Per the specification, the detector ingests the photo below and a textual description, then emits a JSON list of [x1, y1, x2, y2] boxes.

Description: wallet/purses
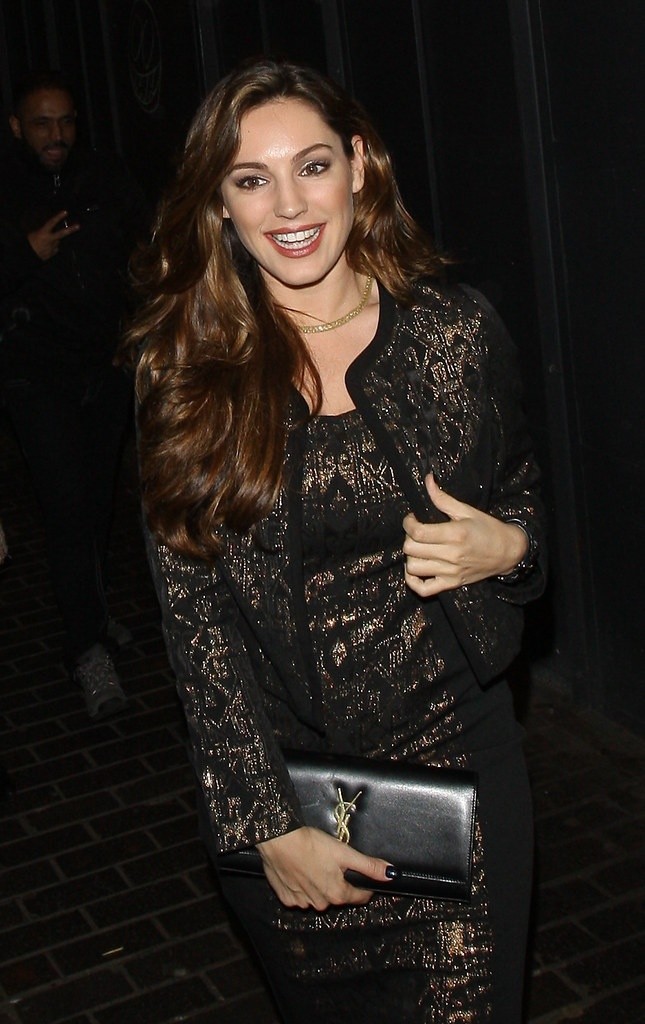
[[213, 750, 480, 906]]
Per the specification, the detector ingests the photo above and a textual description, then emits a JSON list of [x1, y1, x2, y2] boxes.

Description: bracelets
[[498, 517, 536, 584]]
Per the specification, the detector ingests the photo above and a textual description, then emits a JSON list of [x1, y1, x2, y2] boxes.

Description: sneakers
[[72, 640, 134, 724], [105, 616, 140, 649]]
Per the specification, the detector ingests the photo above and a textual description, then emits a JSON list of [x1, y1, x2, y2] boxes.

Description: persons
[[0, 65, 137, 720], [133, 60, 556, 1024]]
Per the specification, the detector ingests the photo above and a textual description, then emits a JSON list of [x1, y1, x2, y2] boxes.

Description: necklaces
[[295, 269, 373, 333]]
